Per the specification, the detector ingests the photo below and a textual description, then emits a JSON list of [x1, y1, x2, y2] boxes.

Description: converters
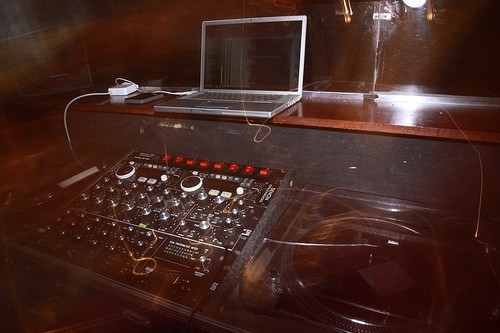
[[108, 83, 139, 96]]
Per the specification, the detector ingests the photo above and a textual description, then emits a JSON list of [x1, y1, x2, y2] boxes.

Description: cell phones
[[124, 90, 164, 104]]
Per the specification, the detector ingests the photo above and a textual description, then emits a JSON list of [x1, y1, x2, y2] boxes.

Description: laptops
[[154, 13, 306, 119]]
